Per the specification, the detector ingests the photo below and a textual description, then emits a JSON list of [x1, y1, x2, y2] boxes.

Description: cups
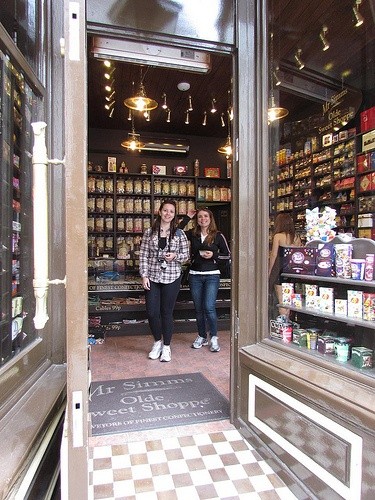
[[350, 259, 365, 280], [283, 323, 293, 342]]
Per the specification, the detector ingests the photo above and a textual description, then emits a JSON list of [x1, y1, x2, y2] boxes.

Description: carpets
[[88, 373, 232, 438]]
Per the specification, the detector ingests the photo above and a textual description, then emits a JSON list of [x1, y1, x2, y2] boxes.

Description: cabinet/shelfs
[[86, 125, 374, 382]]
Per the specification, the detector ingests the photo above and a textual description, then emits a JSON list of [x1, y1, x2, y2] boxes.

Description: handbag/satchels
[[181, 262, 193, 286]]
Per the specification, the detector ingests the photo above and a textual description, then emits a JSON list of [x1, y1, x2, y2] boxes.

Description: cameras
[[158, 259, 168, 269]]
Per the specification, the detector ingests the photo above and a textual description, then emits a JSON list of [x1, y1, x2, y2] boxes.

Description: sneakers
[[209, 336, 220, 352], [192, 334, 208, 348], [159, 344, 171, 362], [148, 339, 163, 359]]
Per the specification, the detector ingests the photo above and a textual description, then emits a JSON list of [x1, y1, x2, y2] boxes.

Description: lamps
[[122, 82, 143, 149], [218, 88, 234, 155], [293, 55, 305, 71], [272, 73, 281, 86], [318, 33, 332, 51], [352, 6, 365, 28], [268, 87, 289, 122], [104, 59, 233, 127]]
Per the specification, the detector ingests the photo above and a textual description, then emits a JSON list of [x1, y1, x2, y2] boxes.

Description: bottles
[[194, 160, 199, 176]]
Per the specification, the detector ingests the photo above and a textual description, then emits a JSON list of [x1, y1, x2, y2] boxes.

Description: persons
[[183, 208, 232, 352], [138, 199, 189, 362], [269, 213, 302, 318]]
